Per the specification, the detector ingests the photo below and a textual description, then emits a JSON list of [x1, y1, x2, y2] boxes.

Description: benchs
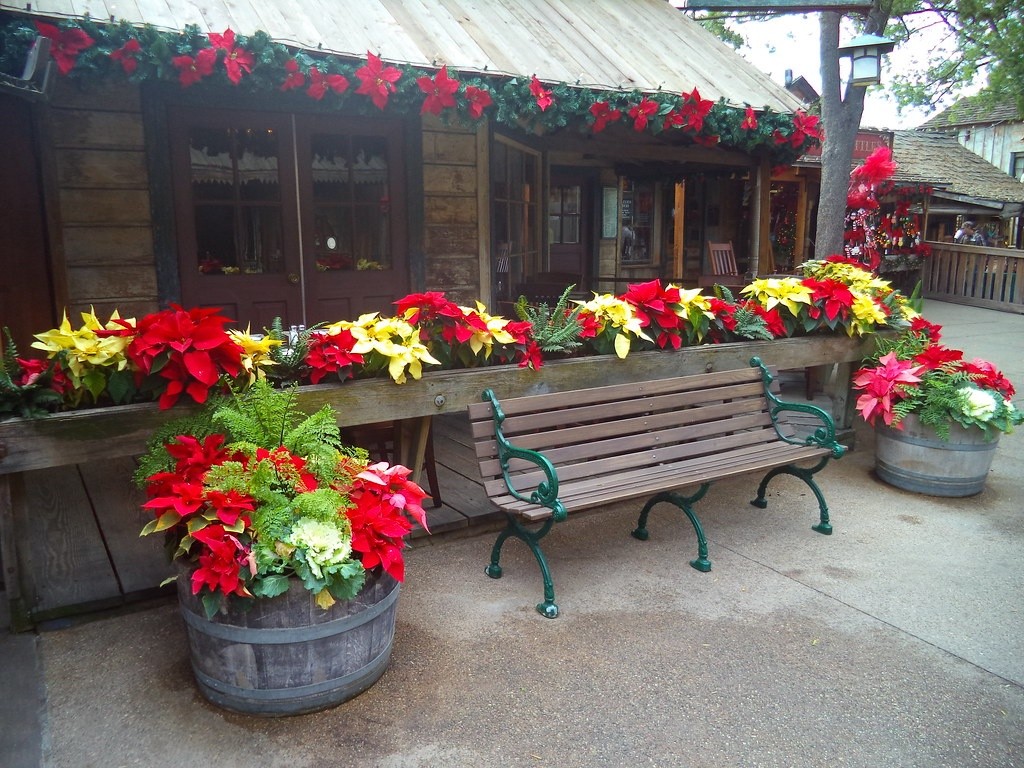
[[468, 355, 848, 619]]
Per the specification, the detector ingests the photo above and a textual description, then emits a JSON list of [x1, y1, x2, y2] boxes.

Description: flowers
[[141, 374, 431, 619], [854, 317, 1024, 443]]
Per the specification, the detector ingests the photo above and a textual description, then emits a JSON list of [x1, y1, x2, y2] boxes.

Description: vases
[[172, 553, 400, 716], [874, 411, 1001, 497]]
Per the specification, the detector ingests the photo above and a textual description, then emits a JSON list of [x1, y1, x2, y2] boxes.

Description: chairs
[[708, 240, 739, 275]]
